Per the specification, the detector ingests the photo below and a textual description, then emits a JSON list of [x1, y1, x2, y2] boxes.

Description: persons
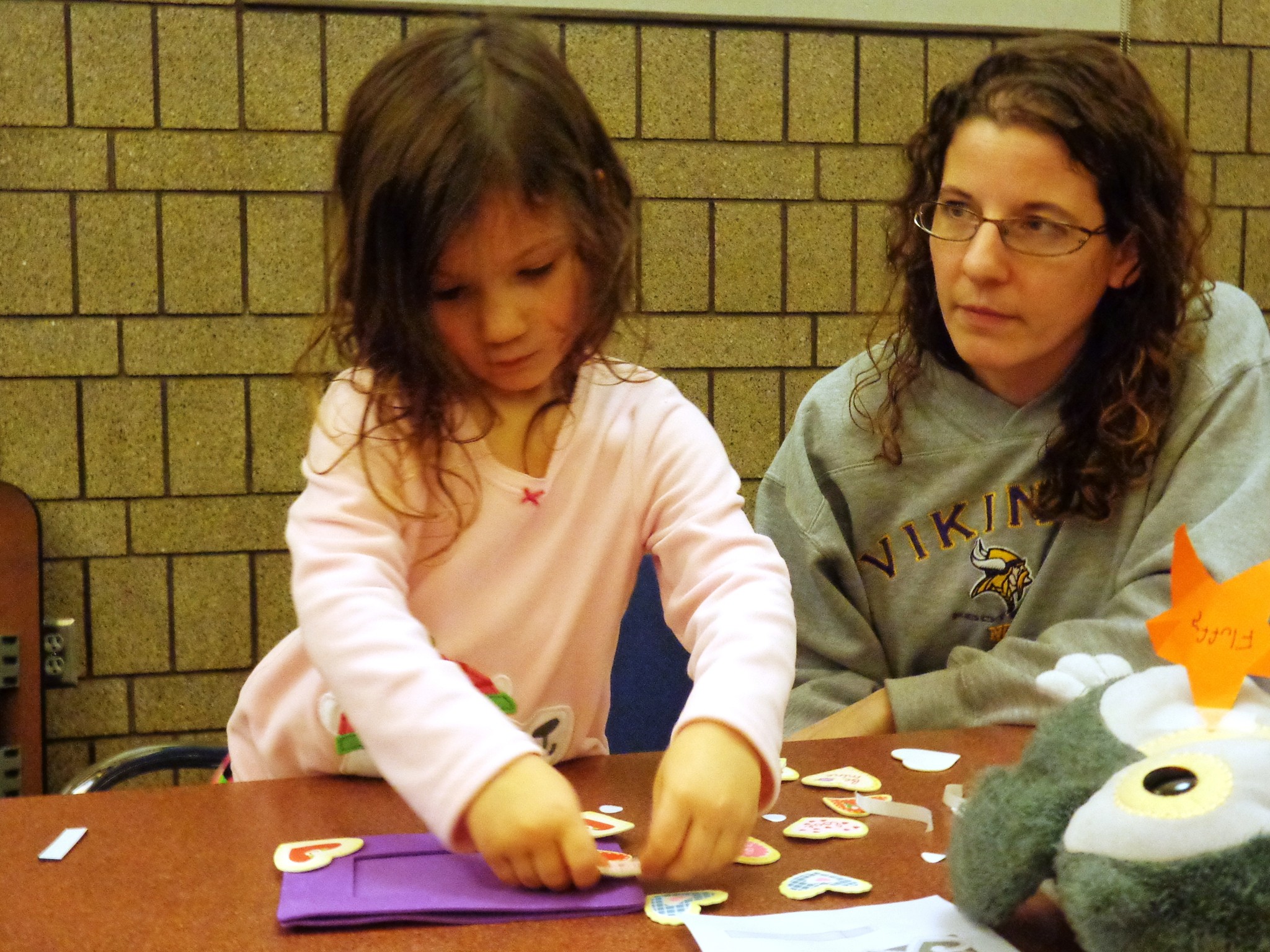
[[754, 38, 1270, 742], [227, 17, 797, 889]]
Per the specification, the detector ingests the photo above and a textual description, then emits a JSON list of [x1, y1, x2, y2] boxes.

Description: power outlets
[[42, 616, 80, 688]]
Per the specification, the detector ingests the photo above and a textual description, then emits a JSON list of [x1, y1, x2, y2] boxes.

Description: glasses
[[913, 198, 1111, 255]]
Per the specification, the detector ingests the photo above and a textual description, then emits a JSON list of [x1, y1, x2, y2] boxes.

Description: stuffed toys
[[947, 665, 1270, 952]]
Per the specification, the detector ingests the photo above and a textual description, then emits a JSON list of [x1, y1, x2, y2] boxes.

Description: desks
[[0, 723, 1072, 952]]
